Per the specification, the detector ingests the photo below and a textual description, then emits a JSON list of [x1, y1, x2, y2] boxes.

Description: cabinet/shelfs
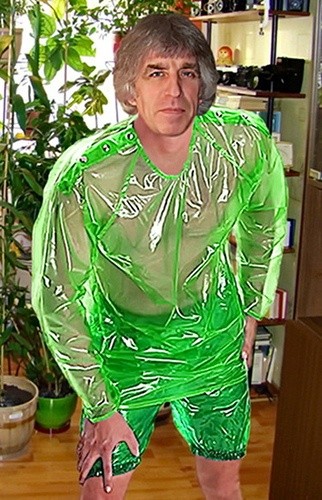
[[188, 10, 311, 402], [269, 0, 322, 500]]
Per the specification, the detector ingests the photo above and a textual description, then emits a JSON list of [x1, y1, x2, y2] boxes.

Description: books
[[210, 79, 295, 384]]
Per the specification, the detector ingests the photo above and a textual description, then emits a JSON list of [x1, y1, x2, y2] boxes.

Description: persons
[[29, 13, 288, 500]]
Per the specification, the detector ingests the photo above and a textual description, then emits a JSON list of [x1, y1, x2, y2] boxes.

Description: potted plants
[[0, 0, 202, 456]]
